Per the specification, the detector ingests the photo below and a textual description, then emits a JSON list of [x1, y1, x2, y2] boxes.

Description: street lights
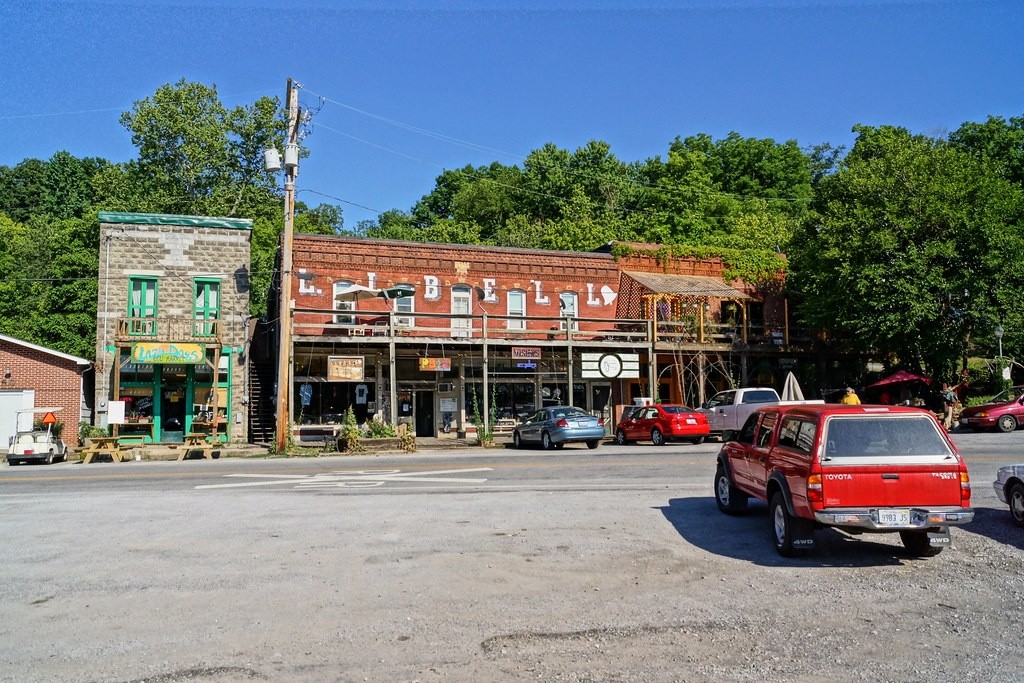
[[994, 322, 1003, 359], [264, 142, 299, 455]]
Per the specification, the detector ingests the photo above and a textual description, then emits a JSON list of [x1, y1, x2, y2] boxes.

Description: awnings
[[623, 271, 751, 298]]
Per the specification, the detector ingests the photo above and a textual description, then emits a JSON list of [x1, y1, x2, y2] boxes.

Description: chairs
[[33, 431, 54, 443], [372, 321, 388, 336], [18, 431, 35, 443], [323, 427, 342, 453]]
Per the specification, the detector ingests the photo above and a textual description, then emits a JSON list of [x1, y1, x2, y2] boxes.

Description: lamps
[[682, 298, 688, 308], [704, 304, 710, 310], [692, 302, 698, 308]]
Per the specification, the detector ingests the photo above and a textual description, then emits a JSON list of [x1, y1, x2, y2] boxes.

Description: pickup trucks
[[695, 387, 825, 443]]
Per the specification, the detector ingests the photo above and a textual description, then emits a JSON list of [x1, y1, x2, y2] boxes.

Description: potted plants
[[129, 410, 138, 423], [457, 428, 466, 439], [217, 410, 225, 422]]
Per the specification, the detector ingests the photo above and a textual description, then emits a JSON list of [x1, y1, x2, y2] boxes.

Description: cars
[[5, 430, 68, 466], [714, 403, 976, 557], [616, 404, 710, 445], [957, 385, 1024, 433], [993, 464, 1024, 529], [513, 405, 605, 451]]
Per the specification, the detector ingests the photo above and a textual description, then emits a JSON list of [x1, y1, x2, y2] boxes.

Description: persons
[[840, 387, 861, 405]]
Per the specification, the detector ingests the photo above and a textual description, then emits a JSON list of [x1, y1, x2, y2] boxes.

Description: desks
[[176, 432, 213, 461], [82, 437, 128, 464]]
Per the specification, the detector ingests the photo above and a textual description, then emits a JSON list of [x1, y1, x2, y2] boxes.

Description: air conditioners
[[438, 383, 453, 394]]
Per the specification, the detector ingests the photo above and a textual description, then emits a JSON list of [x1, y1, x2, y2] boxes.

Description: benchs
[[490, 419, 516, 433], [168, 445, 183, 450], [110, 446, 133, 454], [73, 446, 90, 454], [208, 443, 223, 449]]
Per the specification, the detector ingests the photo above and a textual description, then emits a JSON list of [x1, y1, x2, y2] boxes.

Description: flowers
[[139, 415, 153, 420]]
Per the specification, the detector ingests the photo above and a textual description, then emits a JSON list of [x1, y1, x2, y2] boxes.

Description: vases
[[138, 419, 149, 423]]
